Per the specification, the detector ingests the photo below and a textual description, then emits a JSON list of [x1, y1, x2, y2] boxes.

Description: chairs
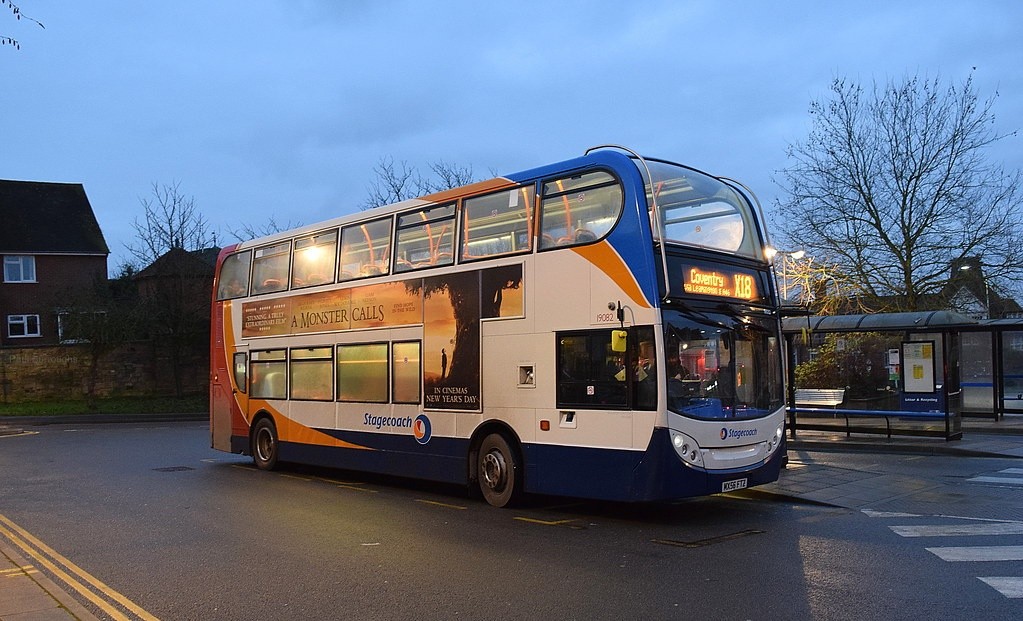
[[221, 227, 596, 299]]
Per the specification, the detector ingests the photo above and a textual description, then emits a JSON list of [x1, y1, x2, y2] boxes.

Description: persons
[[649, 352, 702, 405], [560, 352, 651, 402], [228, 278, 245, 297], [706, 367, 739, 406]]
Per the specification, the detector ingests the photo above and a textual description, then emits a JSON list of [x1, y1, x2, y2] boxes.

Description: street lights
[[958, 263, 994, 383], [762, 245, 805, 386]]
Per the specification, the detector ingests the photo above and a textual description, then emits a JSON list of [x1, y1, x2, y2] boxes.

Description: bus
[[208, 145, 813, 509]]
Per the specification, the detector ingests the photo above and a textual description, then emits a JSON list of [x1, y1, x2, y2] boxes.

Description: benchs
[[791, 388, 845, 420]]
